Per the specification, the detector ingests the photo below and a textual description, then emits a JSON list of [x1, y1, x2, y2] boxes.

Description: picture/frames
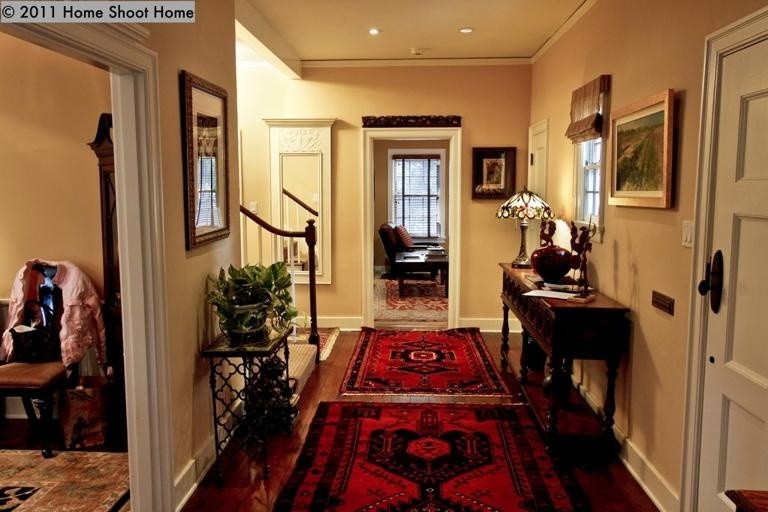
[[607, 88, 676, 209], [178, 70, 230, 251], [471, 145, 517, 200]]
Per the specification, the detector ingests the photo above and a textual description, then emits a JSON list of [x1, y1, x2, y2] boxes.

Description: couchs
[[380, 221, 441, 271]]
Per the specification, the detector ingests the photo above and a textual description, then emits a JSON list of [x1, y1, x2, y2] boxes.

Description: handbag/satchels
[[62, 352, 108, 448]]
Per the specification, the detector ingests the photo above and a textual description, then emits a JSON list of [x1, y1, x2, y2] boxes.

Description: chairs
[[0, 263, 86, 458]]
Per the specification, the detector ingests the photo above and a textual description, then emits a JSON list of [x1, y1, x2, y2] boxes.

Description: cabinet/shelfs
[[200, 325, 296, 487]]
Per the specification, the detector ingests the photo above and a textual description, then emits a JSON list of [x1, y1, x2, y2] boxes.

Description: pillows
[[395, 225, 415, 249]]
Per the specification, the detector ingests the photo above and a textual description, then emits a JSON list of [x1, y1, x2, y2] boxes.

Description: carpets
[[0, 447, 130, 511], [386, 279, 448, 312], [273, 401, 595, 512], [339, 329, 512, 398], [293, 327, 340, 362]]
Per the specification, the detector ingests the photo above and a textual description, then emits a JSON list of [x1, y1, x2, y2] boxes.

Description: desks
[[718, 489, 768, 512], [395, 251, 449, 299], [498, 262, 632, 455]]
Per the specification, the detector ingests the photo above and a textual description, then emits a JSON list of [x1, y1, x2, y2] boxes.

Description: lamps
[[496, 190, 555, 269]]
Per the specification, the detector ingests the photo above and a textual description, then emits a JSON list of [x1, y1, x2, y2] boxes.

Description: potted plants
[[203, 262, 297, 346]]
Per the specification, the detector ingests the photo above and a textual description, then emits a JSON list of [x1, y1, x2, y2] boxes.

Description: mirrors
[[279, 152, 322, 276]]
